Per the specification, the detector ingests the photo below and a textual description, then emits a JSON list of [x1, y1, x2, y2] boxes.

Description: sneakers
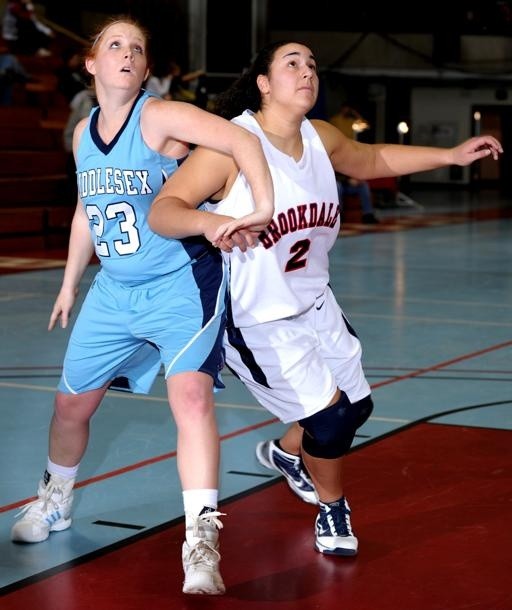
[[314, 498, 359, 557], [181, 517, 226, 596], [255, 438, 320, 505], [10, 470, 75, 544]]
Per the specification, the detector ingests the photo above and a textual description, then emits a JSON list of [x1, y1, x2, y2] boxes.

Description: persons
[[11, 17, 270, 595], [148, 39, 503, 553]]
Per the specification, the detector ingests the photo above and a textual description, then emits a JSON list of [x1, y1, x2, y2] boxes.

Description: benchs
[[0, 23, 86, 254]]
